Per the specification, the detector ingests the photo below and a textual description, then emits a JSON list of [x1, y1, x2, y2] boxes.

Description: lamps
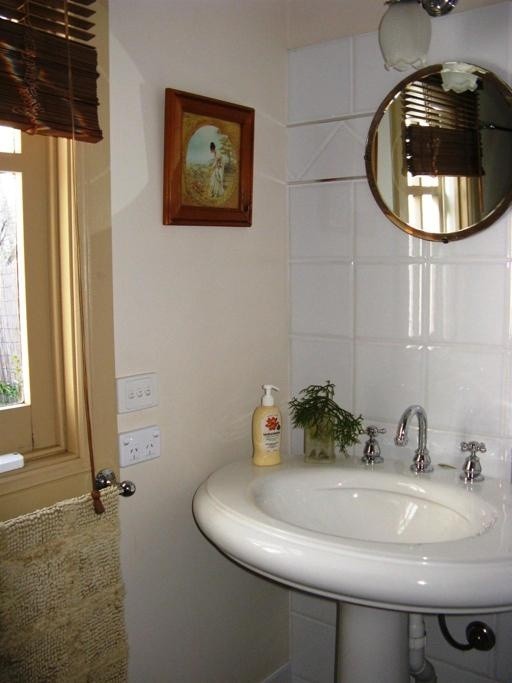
[[377, 0, 458, 72]]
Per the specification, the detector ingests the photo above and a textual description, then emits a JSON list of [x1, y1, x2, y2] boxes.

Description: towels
[[1, 477, 130, 681]]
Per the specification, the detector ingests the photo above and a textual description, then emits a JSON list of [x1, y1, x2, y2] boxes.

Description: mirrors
[[365, 58, 510, 243]]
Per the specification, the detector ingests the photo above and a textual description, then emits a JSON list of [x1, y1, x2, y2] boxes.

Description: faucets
[[394, 405, 434, 473]]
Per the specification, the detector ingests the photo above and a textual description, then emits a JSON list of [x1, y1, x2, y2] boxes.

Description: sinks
[[191, 455, 512, 614]]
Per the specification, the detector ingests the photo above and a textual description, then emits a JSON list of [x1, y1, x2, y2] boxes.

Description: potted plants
[[288, 379, 367, 463]]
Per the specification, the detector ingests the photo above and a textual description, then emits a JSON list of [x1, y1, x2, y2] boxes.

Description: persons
[[208, 141, 227, 196]]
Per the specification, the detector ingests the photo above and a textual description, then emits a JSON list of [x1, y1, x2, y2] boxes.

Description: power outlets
[[117, 425, 161, 468]]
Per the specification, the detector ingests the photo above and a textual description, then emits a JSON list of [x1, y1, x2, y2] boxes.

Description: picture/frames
[[161, 88, 256, 228]]
